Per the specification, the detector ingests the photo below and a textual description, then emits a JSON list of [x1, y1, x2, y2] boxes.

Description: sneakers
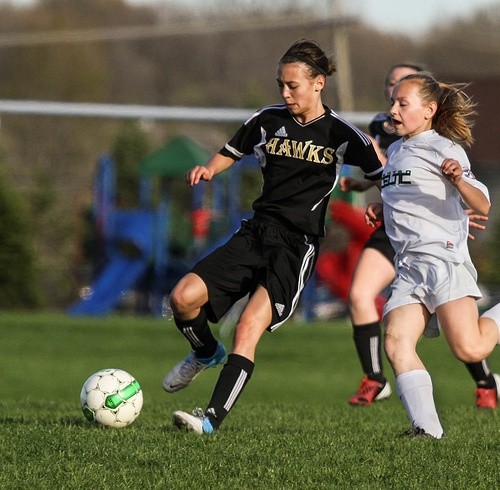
[[171, 407, 209, 435], [475, 372, 500, 406], [162, 341, 227, 392], [349, 374, 394, 406], [400, 420, 448, 442]]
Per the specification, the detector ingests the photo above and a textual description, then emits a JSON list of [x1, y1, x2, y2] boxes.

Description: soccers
[[80, 368, 144, 428]]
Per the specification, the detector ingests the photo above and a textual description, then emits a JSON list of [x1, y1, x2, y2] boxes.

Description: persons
[[365, 72, 500, 440], [161, 38, 487, 434], [340, 64, 500, 409]]
